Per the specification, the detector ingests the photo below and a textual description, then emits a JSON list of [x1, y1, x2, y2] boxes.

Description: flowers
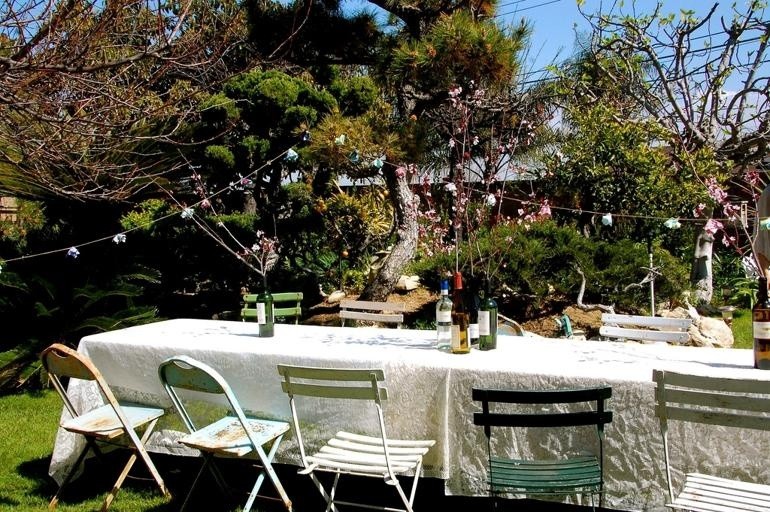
[[668, 119, 770, 279], [387, 78, 563, 282], [150, 146, 293, 276]]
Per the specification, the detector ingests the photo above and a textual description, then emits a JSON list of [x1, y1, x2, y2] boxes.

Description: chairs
[[599, 313, 693, 347], [158, 355, 295, 512], [652, 368, 769, 512], [276, 364, 437, 512], [241, 292, 303, 325], [471, 385, 613, 512], [497, 312, 526, 336], [40, 343, 173, 512], [339, 298, 405, 329]]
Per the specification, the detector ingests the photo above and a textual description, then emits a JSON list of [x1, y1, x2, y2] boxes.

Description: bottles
[[255, 280, 275, 338], [450, 272, 470, 355], [435, 280, 453, 352], [477, 278, 498, 350], [751, 277, 770, 371]]
